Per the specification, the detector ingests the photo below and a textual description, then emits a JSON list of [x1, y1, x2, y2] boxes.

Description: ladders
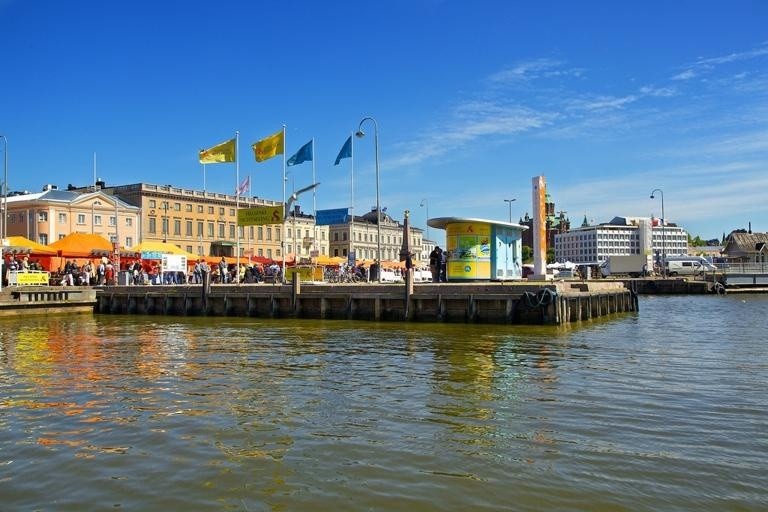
[[112, 242, 120, 285]]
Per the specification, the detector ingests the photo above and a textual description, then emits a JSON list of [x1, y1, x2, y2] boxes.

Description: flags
[[199, 137, 236, 164], [333, 135, 352, 164], [235, 176, 250, 197], [286, 140, 314, 166], [253, 130, 284, 162]]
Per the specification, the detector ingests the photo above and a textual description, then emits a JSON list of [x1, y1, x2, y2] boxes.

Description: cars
[[521, 266, 534, 278], [554, 270, 576, 278]]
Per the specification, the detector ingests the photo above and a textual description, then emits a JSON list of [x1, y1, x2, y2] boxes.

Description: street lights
[[354, 114, 381, 283], [419, 197, 429, 266], [283, 170, 297, 261], [0, 135, 7, 238], [503, 198, 516, 223], [648, 187, 665, 277], [0, 180, 10, 238]]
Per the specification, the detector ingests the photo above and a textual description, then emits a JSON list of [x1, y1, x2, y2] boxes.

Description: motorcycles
[[330, 257, 369, 282]]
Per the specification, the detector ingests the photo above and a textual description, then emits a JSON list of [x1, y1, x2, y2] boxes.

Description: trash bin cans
[[118, 271, 129, 286], [369, 264, 380, 284]]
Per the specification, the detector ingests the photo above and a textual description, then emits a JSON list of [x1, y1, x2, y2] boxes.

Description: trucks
[[703, 255, 730, 268], [666, 260, 709, 277], [598, 253, 647, 278], [661, 254, 718, 273]]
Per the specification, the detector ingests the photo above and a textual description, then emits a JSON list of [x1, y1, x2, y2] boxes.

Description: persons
[[0, 252, 281, 284], [325, 259, 377, 282], [429, 246, 447, 283]]
